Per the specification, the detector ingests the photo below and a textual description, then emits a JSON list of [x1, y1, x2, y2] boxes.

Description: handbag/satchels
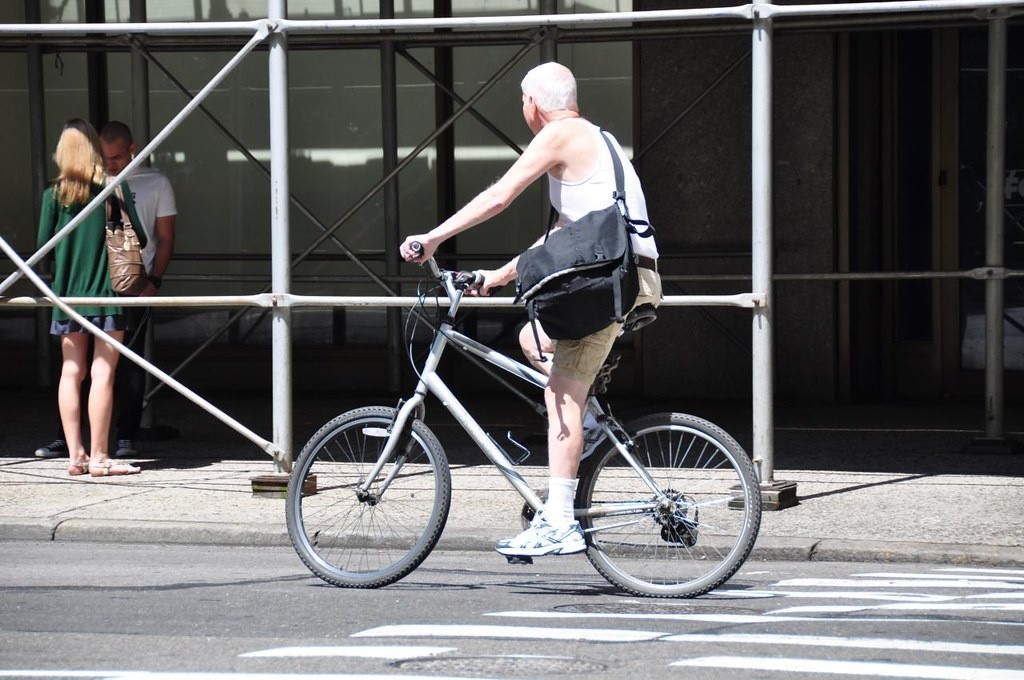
[[104, 177, 147, 295], [514, 203, 655, 341]]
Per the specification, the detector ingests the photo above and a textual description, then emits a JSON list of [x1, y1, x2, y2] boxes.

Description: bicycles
[[283, 243, 763, 598]]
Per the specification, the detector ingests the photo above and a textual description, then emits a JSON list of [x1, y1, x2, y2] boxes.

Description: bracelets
[[149, 276, 161, 289]]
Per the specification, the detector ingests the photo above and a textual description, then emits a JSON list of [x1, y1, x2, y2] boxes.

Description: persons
[[399, 62, 662, 558], [34, 119, 181, 458], [37, 122, 147, 476]]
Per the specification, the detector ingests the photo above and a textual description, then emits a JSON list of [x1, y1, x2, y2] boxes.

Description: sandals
[[68, 461, 90, 476], [88, 462, 141, 477]]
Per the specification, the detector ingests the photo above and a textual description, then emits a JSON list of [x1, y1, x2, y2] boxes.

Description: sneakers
[[35, 438, 68, 458], [495, 519, 588, 556], [578, 416, 622, 460], [114, 438, 139, 456]]
